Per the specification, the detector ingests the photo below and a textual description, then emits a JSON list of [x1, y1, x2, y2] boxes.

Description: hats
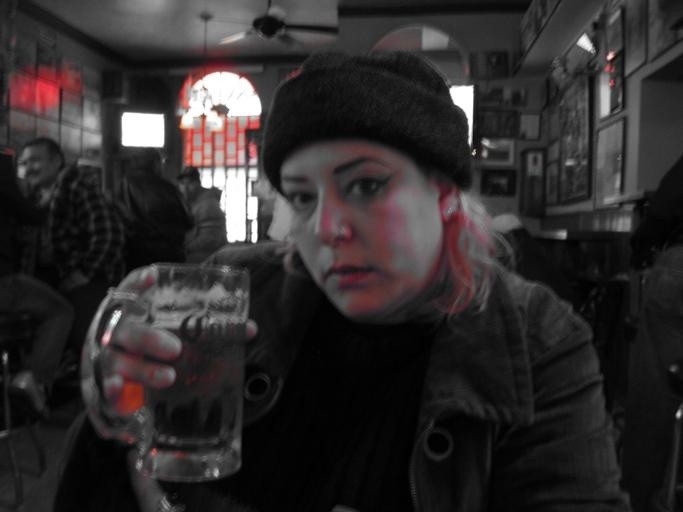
[[262, 49, 470, 192]]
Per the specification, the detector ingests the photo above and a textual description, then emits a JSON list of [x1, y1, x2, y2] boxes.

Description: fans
[[210, 1, 339, 49]]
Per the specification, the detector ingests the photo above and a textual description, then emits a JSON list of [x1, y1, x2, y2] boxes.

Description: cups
[[79, 264, 250, 482]]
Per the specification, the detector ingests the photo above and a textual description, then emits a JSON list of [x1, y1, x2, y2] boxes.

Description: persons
[[52, 50, 628, 512], [488, 210, 548, 284], [116, 144, 225, 271], [0, 137, 124, 416], [612, 152, 683, 498]]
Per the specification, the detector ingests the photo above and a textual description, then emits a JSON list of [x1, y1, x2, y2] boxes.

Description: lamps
[[182, 12, 224, 132]]
